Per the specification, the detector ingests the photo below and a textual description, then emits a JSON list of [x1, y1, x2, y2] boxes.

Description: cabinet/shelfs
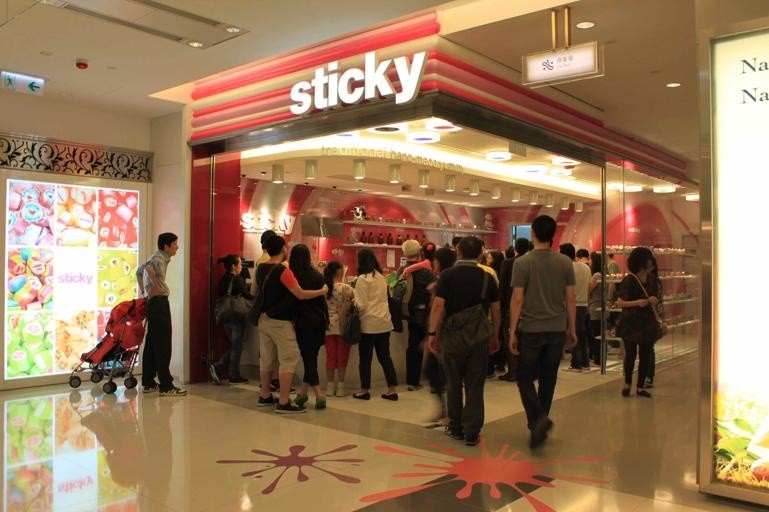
[[596, 251, 699, 340], [344, 220, 499, 254]]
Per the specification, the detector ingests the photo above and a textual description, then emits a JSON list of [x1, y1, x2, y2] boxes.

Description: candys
[[6, 182, 139, 380], [7, 388, 139, 512]]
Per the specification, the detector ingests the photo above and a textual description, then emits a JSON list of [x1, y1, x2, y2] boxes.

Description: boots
[[325, 381, 350, 398]]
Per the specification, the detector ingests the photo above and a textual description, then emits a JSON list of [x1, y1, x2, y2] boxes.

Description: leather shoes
[[380, 392, 398, 402], [352, 391, 372, 401]]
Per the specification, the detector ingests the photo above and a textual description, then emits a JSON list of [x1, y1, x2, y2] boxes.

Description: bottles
[[360, 231, 428, 248]]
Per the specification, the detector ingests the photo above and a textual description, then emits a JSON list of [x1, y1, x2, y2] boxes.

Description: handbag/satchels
[[443, 304, 493, 352], [649, 310, 668, 338], [245, 288, 267, 326], [342, 303, 362, 345], [387, 298, 404, 334], [215, 292, 255, 326]]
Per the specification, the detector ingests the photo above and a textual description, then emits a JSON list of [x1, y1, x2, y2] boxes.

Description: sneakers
[[274, 394, 309, 414], [621, 377, 656, 398], [463, 434, 480, 445], [408, 363, 517, 395], [258, 379, 280, 406], [444, 425, 464, 440], [530, 413, 554, 451], [144, 384, 159, 394], [563, 351, 602, 373], [315, 396, 327, 409], [208, 363, 223, 385], [158, 385, 188, 396], [228, 375, 249, 384]]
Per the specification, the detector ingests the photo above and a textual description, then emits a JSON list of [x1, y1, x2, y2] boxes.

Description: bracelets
[[141, 290, 144, 294]]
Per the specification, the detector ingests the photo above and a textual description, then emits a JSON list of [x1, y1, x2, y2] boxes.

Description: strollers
[[65, 387, 152, 490], [69, 298, 148, 394]]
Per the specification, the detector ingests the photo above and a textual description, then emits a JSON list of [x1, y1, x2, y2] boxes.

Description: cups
[[366, 214, 488, 231]]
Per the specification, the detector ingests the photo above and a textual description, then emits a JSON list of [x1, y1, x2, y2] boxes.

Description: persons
[[615, 247, 663, 397], [559, 243, 619, 372], [249, 230, 355, 412], [400, 235, 535, 444], [507, 214, 577, 451], [136, 232, 187, 396], [349, 248, 399, 401], [210, 254, 255, 383]]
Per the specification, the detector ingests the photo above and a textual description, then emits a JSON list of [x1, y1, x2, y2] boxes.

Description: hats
[[401, 239, 420, 257], [389, 271, 414, 304], [423, 241, 436, 262]]
